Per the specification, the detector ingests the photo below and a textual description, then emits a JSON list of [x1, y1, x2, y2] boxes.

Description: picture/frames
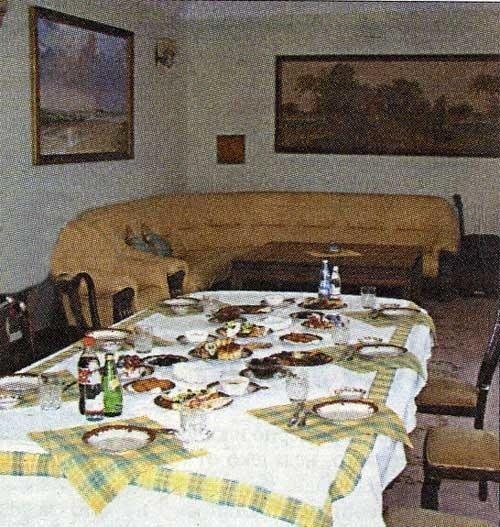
[[27, 5, 135, 168], [274, 53, 498, 159], [215, 132, 245, 166]]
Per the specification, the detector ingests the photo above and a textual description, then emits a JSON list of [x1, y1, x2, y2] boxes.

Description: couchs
[[49, 189, 463, 329]]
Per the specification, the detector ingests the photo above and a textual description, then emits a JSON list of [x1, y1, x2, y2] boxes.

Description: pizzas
[[157, 391, 230, 409]]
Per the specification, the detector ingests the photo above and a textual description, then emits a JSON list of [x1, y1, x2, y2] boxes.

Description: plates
[[82, 424, 156, 455], [355, 341, 408, 359], [0, 371, 47, 396], [126, 292, 381, 441], [81, 325, 131, 344], [376, 306, 419, 319]]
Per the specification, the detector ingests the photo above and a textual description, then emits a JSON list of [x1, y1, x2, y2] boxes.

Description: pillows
[[141, 227, 172, 256], [125, 222, 150, 254]]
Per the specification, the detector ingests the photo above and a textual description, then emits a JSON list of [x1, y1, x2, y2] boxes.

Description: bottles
[[75, 338, 100, 414], [318, 259, 331, 297], [329, 264, 343, 300], [102, 352, 124, 417]]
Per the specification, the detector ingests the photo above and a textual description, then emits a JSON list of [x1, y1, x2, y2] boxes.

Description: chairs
[[5, 301, 33, 368], [421, 422, 498, 514], [414, 308, 495, 499], [385, 504, 500, 527]]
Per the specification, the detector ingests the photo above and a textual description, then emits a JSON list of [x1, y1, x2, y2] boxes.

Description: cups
[[82, 383, 104, 422], [38, 373, 61, 410], [360, 285, 377, 309]]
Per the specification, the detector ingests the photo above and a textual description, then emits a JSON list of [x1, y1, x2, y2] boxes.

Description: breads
[[194, 337, 243, 361], [134, 378, 170, 392]]
